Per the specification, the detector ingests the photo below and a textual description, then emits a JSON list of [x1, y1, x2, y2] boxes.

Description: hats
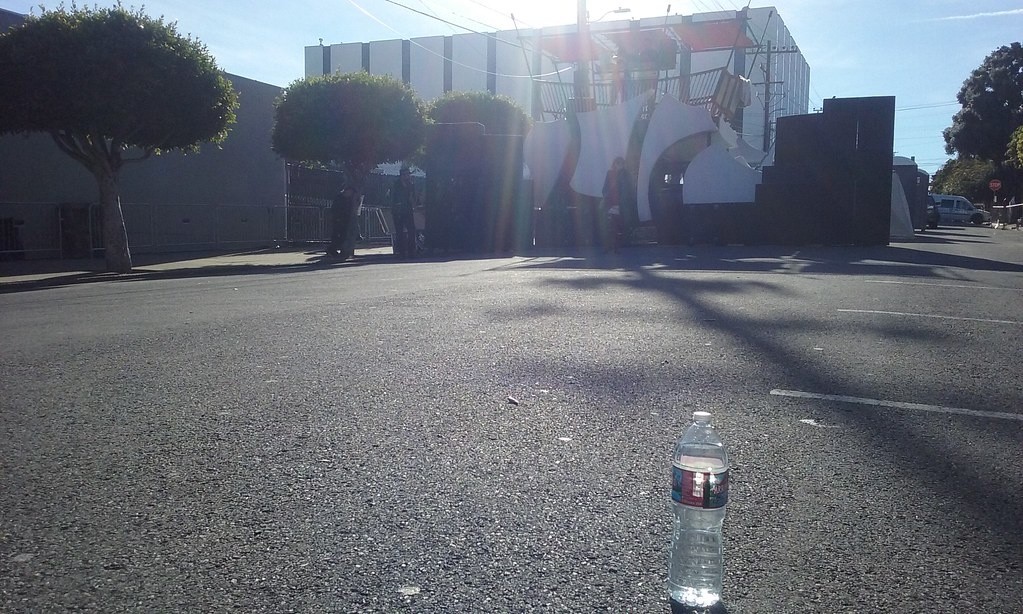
[[395, 168, 415, 177]]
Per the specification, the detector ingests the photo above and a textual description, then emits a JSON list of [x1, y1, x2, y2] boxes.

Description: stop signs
[[989, 179, 1002, 191]]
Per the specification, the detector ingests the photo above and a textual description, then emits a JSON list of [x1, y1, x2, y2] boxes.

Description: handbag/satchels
[[599, 195, 607, 210]]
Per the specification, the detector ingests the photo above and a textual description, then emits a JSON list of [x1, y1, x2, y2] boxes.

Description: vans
[[932, 194, 991, 225]]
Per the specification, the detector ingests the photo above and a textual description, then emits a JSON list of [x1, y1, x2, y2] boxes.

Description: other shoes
[[408, 253, 421, 259], [399, 255, 406, 260]]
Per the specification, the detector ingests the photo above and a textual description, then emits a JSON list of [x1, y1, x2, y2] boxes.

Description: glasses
[[616, 162, 624, 165]]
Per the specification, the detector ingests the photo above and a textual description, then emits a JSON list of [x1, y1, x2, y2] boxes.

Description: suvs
[[923, 195, 941, 228]]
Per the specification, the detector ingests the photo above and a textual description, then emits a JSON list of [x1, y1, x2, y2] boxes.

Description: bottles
[[668, 411, 729, 607]]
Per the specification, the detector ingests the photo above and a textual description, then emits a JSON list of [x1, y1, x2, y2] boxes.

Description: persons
[[601, 156, 631, 252], [330, 187, 356, 255], [390, 168, 422, 259]]
